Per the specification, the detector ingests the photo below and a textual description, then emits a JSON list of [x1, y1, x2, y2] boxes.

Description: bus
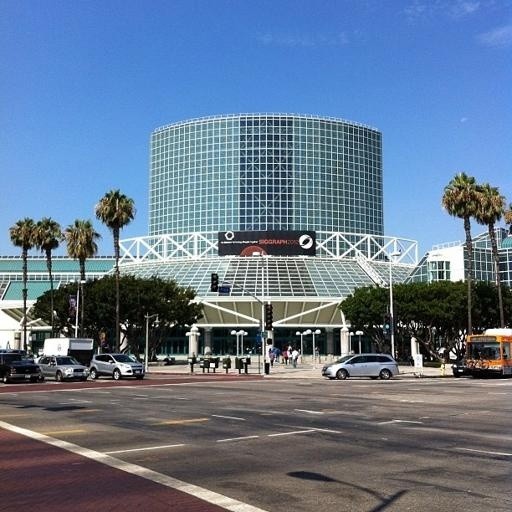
[[466, 328, 512, 379]]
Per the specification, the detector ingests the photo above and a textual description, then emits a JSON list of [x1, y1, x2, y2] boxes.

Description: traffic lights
[[211, 273, 219, 292], [394, 314, 401, 335], [266, 339, 273, 345], [383, 314, 390, 334], [264, 303, 274, 330]]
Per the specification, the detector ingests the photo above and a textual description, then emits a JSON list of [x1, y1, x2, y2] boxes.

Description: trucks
[[43, 337, 94, 367]]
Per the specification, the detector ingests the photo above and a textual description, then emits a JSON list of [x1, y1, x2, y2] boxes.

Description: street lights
[[231, 329, 248, 356], [346, 330, 364, 354], [144, 312, 161, 373], [296, 329, 321, 362], [75, 277, 87, 338], [390, 251, 402, 359]]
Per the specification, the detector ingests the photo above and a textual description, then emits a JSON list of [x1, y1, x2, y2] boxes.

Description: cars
[[452, 357, 466, 377], [0, 349, 91, 383], [322, 353, 399, 380], [89, 352, 145, 380]]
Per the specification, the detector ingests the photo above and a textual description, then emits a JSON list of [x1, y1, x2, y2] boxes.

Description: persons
[[269, 344, 301, 367]]
